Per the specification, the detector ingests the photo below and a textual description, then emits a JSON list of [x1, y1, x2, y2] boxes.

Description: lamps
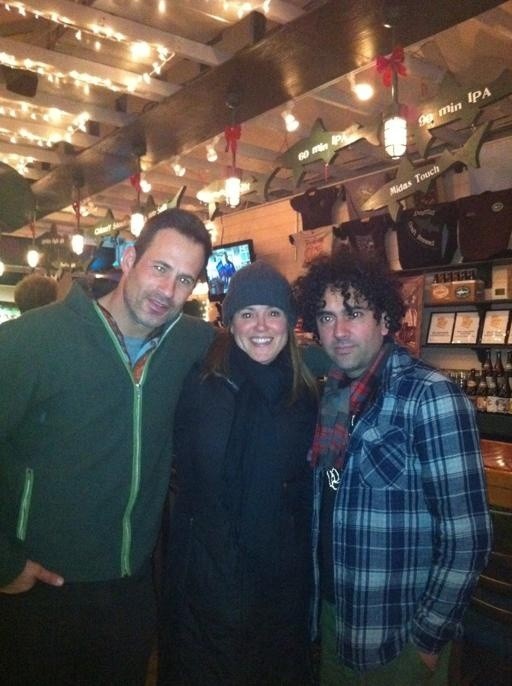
[[25, 16, 407, 268]]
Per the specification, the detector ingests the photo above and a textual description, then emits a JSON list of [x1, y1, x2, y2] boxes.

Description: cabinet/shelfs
[[394, 257, 512, 444]]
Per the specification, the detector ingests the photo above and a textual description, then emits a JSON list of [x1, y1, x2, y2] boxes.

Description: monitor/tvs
[[204, 240, 256, 301]]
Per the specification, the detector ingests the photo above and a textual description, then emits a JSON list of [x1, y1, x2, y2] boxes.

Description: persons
[[14, 275, 57, 314], [0, 208, 332, 686], [291, 248, 495, 686], [153, 259, 318, 686]]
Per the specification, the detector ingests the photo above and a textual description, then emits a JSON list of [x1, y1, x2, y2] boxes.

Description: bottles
[[433, 347, 512, 413], [433, 269, 475, 282]]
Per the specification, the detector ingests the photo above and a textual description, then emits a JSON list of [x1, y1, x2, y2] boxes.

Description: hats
[[222, 259, 297, 327]]
